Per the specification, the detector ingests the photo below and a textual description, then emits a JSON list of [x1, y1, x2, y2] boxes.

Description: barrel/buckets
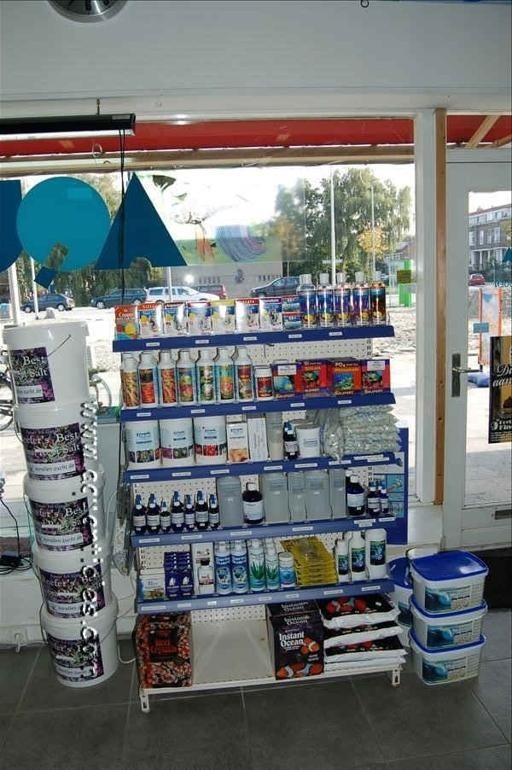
[[33, 550, 113, 615], [40, 602, 119, 687], [23, 481, 99, 560], [13, 408, 95, 480], [1, 321, 91, 406]]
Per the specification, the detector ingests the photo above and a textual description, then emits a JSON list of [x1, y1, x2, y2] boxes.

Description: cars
[[20, 293, 77, 313], [380, 273, 397, 287], [468, 272, 485, 285]]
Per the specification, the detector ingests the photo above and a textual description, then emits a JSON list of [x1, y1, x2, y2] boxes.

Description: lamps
[[0, 96, 138, 140]]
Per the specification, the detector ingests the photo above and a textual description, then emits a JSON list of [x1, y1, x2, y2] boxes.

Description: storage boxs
[[130, 611, 194, 692], [265, 602, 324, 683], [387, 542, 490, 692]]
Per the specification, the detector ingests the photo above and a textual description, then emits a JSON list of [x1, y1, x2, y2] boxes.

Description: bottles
[[197, 558, 215, 595], [295, 270, 387, 331], [129, 489, 218, 538], [283, 422, 298, 462], [213, 540, 278, 596], [117, 346, 253, 411], [279, 552, 297, 591], [334, 528, 386, 580], [343, 468, 391, 515]]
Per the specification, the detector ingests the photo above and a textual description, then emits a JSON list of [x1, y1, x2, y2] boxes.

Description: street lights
[[369, 168, 384, 282]]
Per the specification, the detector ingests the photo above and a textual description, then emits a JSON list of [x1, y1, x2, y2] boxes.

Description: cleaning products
[[284, 420, 299, 460], [214, 539, 280, 595], [368, 485, 390, 515], [119, 346, 255, 408], [297, 270, 386, 329], [336, 529, 387, 582], [345, 475, 365, 516]]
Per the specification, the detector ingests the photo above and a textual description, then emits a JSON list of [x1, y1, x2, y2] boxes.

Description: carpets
[[465, 548, 511, 613]]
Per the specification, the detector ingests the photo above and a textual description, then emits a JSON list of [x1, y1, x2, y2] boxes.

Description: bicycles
[[0, 349, 112, 434]]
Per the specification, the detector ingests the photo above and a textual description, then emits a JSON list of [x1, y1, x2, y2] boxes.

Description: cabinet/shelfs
[[114, 308, 411, 713]]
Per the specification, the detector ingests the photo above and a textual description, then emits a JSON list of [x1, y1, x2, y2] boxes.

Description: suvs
[[91, 287, 148, 309], [250, 277, 302, 299], [145, 287, 221, 305], [194, 285, 227, 300]]
